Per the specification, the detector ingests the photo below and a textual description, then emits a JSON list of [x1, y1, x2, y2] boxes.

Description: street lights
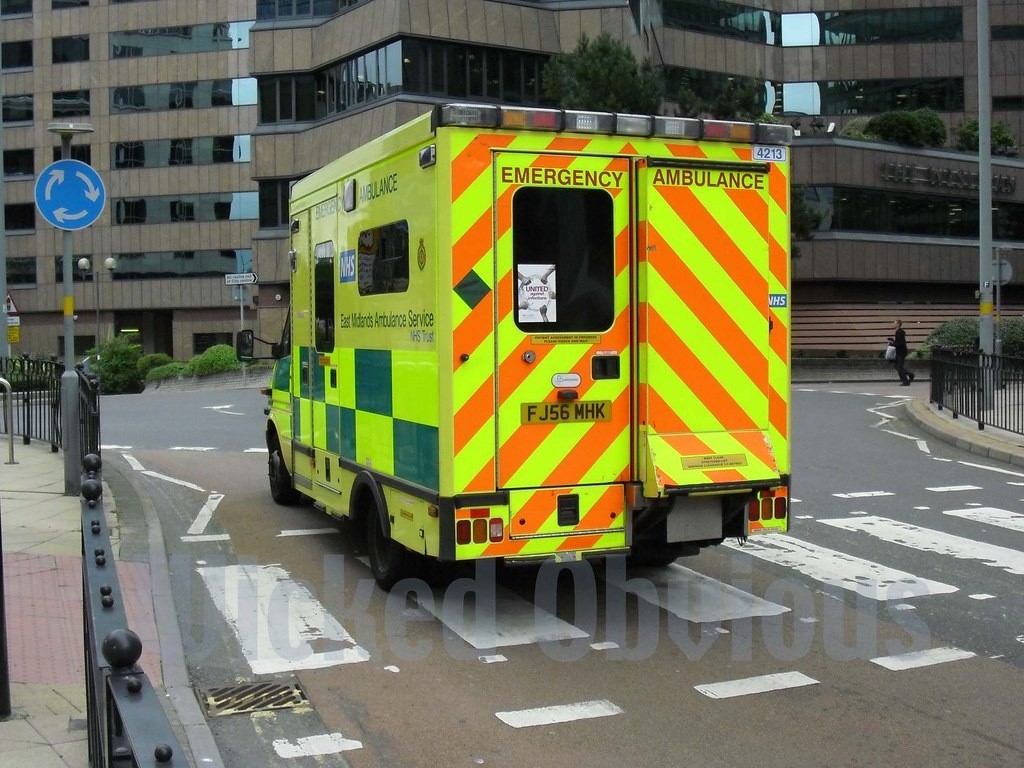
[[79, 256, 118, 355]]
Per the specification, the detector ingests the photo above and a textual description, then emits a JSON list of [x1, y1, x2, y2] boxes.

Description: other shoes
[[909, 373, 915, 382], [899, 382, 910, 386]]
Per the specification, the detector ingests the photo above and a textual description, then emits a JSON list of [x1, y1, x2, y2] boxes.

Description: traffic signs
[[225, 272, 258, 285]]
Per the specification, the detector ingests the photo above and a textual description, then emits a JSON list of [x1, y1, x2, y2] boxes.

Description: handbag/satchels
[[885, 346, 896, 359]]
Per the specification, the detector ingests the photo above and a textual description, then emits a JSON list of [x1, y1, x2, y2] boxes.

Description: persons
[[888, 320, 915, 386]]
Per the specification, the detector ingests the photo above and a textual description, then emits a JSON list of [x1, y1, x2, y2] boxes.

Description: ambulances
[[235, 102, 793, 592]]
